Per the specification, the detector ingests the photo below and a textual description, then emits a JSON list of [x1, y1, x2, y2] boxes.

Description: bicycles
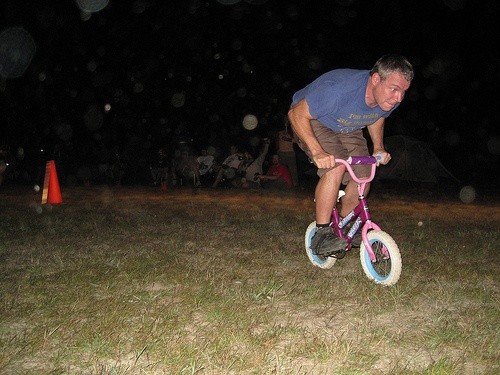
[[306, 154, 403, 285]]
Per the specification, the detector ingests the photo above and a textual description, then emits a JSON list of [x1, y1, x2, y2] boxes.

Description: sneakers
[[341, 223, 362, 247], [310, 226, 348, 256]]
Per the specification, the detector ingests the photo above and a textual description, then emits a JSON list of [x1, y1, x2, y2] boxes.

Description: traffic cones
[[42, 160, 63, 204]]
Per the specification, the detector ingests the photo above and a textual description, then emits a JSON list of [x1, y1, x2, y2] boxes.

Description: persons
[[288, 54, 414, 254], [145, 118, 293, 195]]
